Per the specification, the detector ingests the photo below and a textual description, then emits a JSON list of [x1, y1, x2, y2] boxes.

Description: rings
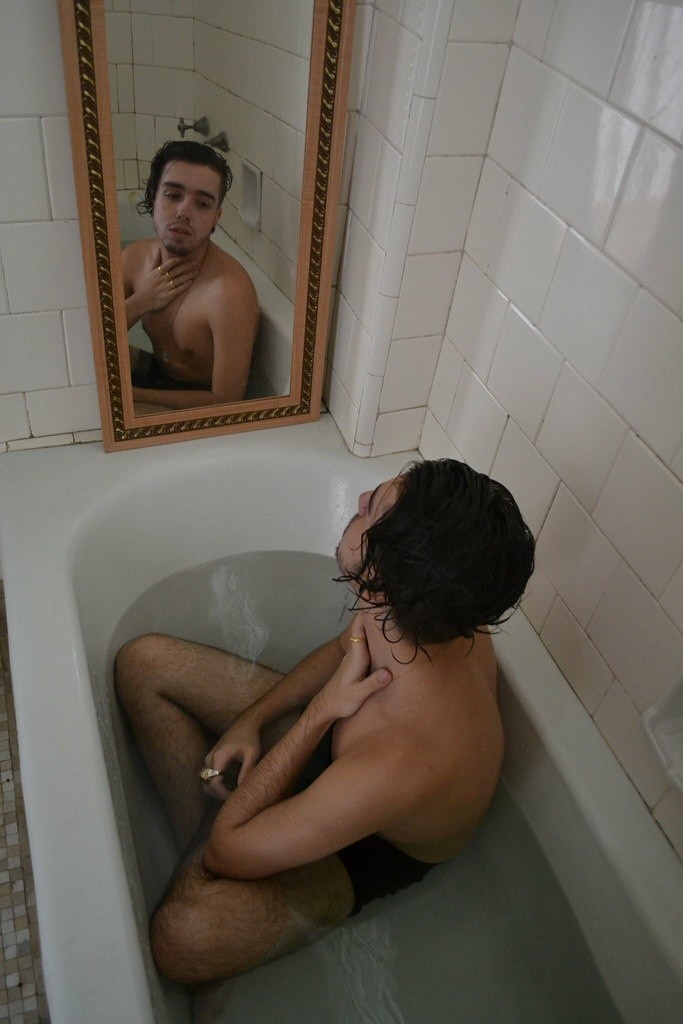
[[198, 765, 223, 784], [158, 266, 163, 273], [169, 280, 175, 289], [350, 635, 367, 646], [166, 271, 171, 279]]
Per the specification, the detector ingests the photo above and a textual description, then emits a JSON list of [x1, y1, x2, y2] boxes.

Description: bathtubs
[[1, 411, 682, 1023], [113, 185, 294, 417]]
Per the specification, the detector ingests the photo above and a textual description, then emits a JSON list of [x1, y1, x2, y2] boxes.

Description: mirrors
[[57, 0, 354, 452]]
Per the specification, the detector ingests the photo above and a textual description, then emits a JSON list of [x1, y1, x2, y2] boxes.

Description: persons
[[121, 140, 260, 420], [111, 456, 537, 991]]
